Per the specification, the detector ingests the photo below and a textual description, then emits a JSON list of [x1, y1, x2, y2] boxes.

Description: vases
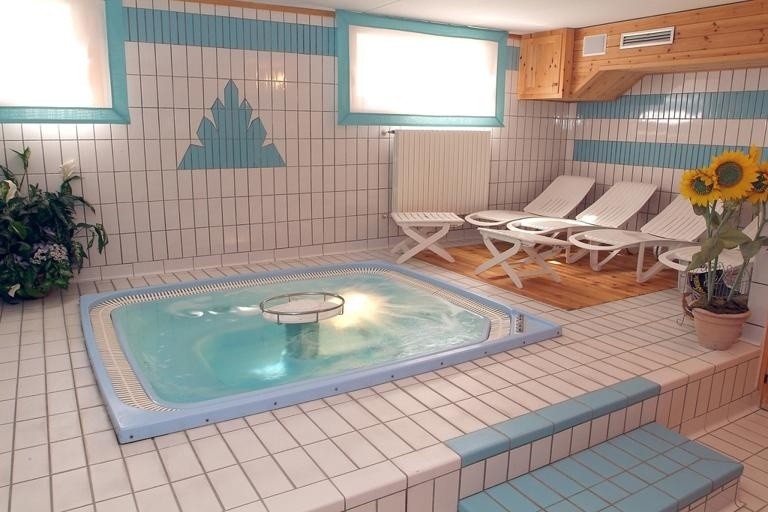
[[688, 300, 752, 351], [32, 271, 48, 298]]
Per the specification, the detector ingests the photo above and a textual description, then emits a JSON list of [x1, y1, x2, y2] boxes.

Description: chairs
[[464, 176, 595, 226], [568, 194, 724, 282], [507, 182, 658, 264], [658, 216, 758, 292]]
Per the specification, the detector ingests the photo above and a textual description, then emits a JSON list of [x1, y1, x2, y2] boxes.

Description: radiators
[[382, 130, 491, 218]]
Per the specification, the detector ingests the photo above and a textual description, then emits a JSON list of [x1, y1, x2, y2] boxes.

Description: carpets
[[414, 242, 678, 312]]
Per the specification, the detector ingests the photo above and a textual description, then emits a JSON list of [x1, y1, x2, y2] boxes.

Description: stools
[[475, 227, 574, 289], [390, 212, 464, 264]]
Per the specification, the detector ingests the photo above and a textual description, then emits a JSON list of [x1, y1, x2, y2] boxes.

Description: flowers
[[0, 148, 108, 304], [681, 145, 768, 304]]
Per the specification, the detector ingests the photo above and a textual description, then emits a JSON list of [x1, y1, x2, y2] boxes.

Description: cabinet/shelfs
[[518, 29, 574, 101]]
